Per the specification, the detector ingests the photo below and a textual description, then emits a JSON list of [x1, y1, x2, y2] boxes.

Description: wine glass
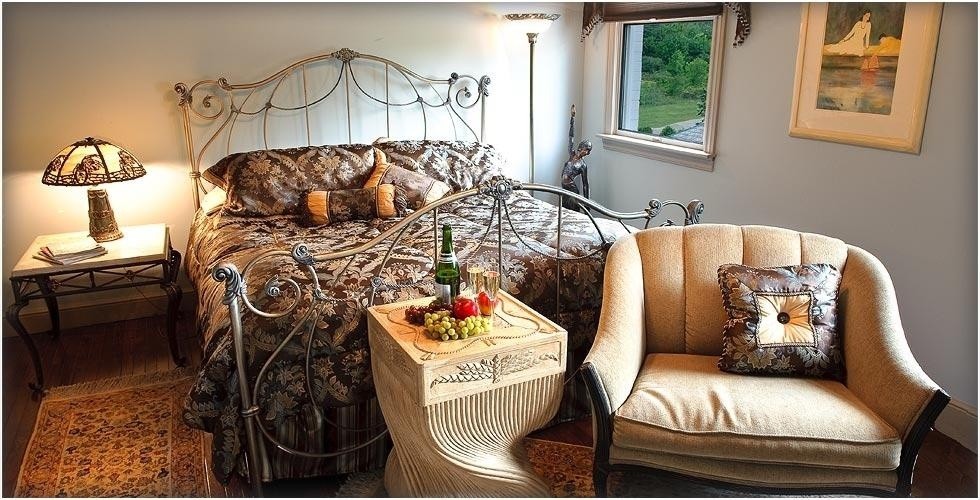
[[466, 259, 502, 326]]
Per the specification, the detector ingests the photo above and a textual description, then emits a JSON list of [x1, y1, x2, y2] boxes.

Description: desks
[[4, 221, 193, 402], [368, 289, 571, 497]]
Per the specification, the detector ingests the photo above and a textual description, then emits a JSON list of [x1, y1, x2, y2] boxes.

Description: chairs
[[582, 222, 955, 497]]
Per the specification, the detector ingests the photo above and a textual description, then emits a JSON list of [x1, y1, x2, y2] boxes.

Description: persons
[[561, 104, 592, 215], [874, 33, 901, 55], [824, 9, 872, 57]]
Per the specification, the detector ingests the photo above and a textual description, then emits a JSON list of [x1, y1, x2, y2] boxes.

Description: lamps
[[42, 134, 147, 243], [503, 11, 563, 198]]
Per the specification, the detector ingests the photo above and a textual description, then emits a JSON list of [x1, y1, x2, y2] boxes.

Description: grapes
[[405, 300, 454, 325], [424, 310, 492, 341]]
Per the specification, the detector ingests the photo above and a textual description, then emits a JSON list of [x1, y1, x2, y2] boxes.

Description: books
[[33, 236, 108, 266]]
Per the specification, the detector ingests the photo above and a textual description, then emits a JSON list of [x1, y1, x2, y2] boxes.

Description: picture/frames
[[786, 2, 945, 157]]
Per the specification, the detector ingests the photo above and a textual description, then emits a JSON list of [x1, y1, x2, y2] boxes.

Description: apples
[[478, 291, 499, 316], [453, 298, 478, 320]]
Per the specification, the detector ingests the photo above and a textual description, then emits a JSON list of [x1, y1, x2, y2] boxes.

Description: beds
[[171, 46, 704, 497]]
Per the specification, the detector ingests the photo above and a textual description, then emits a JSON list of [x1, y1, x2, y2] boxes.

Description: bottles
[[434, 222, 460, 303]]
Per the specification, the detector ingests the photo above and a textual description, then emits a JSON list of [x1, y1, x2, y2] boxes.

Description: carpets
[[15, 366, 229, 495], [329, 423, 704, 497]]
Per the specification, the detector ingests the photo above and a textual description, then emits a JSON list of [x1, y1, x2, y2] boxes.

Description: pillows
[[363, 157, 461, 219], [301, 182, 407, 230], [378, 132, 532, 198], [712, 256, 849, 385], [203, 140, 385, 227]]
